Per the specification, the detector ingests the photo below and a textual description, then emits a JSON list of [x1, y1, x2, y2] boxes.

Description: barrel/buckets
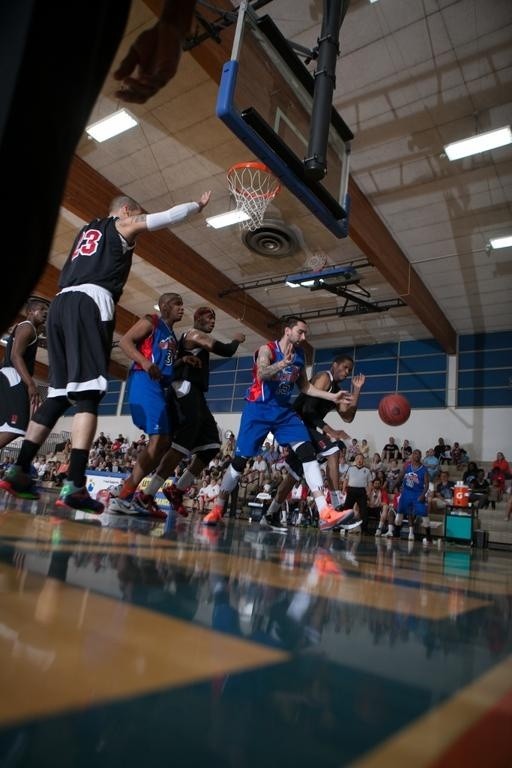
[[452, 484, 470, 508]]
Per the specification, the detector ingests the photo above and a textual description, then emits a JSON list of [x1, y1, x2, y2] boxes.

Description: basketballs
[[380, 395, 409, 425]]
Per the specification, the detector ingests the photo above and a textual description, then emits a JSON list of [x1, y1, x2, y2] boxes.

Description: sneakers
[[375, 527, 401, 539], [318, 503, 363, 531], [202, 504, 224, 526], [408, 535, 415, 540], [260, 512, 289, 531], [1, 465, 40, 500], [54, 481, 188, 519]]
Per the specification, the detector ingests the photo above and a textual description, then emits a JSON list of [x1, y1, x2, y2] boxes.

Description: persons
[[0, 190, 212, 513], [1, 456, 14, 472], [34, 439, 72, 486], [107, 293, 201, 513], [136, 306, 245, 518], [261, 356, 365, 528], [174, 430, 512, 540], [88, 432, 146, 472], [0, 300, 47, 474], [204, 317, 352, 529]]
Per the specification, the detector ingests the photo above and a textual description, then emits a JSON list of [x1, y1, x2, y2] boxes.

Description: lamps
[[485, 203, 512, 257], [205, 191, 250, 230], [86, 76, 138, 144], [285, 279, 325, 289], [440, 112, 512, 163]]
